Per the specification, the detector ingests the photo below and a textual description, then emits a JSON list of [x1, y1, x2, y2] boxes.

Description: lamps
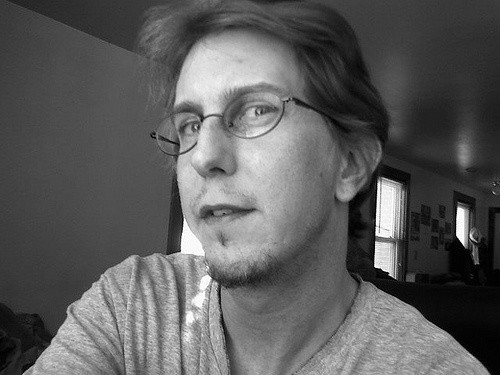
[[492, 181, 500, 199]]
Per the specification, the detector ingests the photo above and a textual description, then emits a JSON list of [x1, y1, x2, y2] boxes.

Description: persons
[[20, 0, 490, 375]]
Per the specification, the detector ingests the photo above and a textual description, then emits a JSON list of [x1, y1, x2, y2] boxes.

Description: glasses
[[149, 90, 352, 157]]
